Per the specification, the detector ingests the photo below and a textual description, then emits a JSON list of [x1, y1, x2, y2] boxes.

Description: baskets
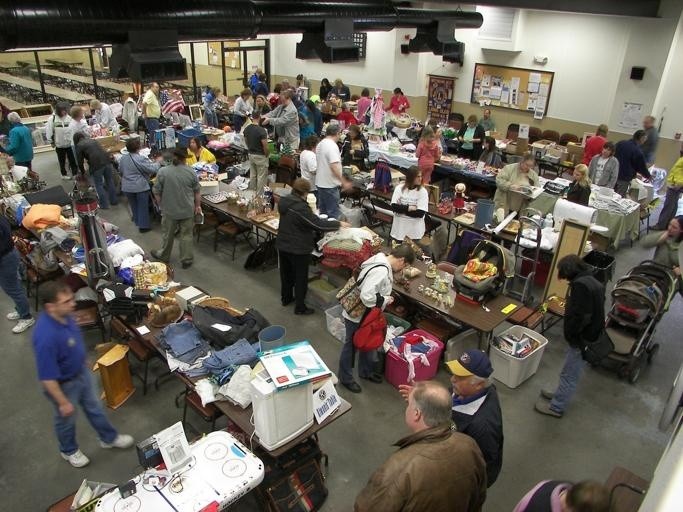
[[396, 113, 411, 128]]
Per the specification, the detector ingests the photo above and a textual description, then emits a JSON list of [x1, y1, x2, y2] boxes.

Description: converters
[[119, 480, 137, 499]]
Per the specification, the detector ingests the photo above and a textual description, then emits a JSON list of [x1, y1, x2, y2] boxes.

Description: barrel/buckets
[[257, 325, 287, 352], [474, 199, 496, 228]]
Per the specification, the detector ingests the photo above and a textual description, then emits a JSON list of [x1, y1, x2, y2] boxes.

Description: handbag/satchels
[[578, 330, 614, 364], [336, 276, 366, 318]]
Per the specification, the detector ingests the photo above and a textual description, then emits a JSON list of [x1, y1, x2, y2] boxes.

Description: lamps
[[1, 4, 483, 80]]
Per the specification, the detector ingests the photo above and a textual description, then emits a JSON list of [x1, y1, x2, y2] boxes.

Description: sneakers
[[541, 388, 555, 399], [139, 228, 151, 232], [282, 297, 294, 306], [360, 374, 382, 383], [294, 309, 313, 315], [536, 403, 562, 417], [61, 450, 89, 467], [340, 379, 362, 392], [100, 434, 133, 449], [150, 250, 168, 262], [8, 313, 21, 319], [12, 318, 35, 333], [183, 262, 191, 268]]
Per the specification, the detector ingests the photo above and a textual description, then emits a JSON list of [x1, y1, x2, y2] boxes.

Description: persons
[[515, 478, 610, 511], [31, 282, 135, 467], [1, 66, 683, 418], [353, 350, 503, 512]]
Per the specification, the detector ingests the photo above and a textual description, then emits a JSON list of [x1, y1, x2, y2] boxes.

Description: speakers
[[629, 66, 646, 81], [400, 43, 410, 54]]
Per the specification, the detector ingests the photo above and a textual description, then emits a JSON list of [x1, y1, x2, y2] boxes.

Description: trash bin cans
[[582, 249, 616, 301]]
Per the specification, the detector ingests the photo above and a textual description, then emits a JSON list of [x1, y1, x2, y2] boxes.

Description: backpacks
[[354, 292, 387, 351]]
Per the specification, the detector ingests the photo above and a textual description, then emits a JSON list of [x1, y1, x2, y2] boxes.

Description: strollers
[[588, 259, 677, 384]]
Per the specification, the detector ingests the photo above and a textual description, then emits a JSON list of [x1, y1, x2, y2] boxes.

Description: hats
[[445, 350, 493, 378]]
